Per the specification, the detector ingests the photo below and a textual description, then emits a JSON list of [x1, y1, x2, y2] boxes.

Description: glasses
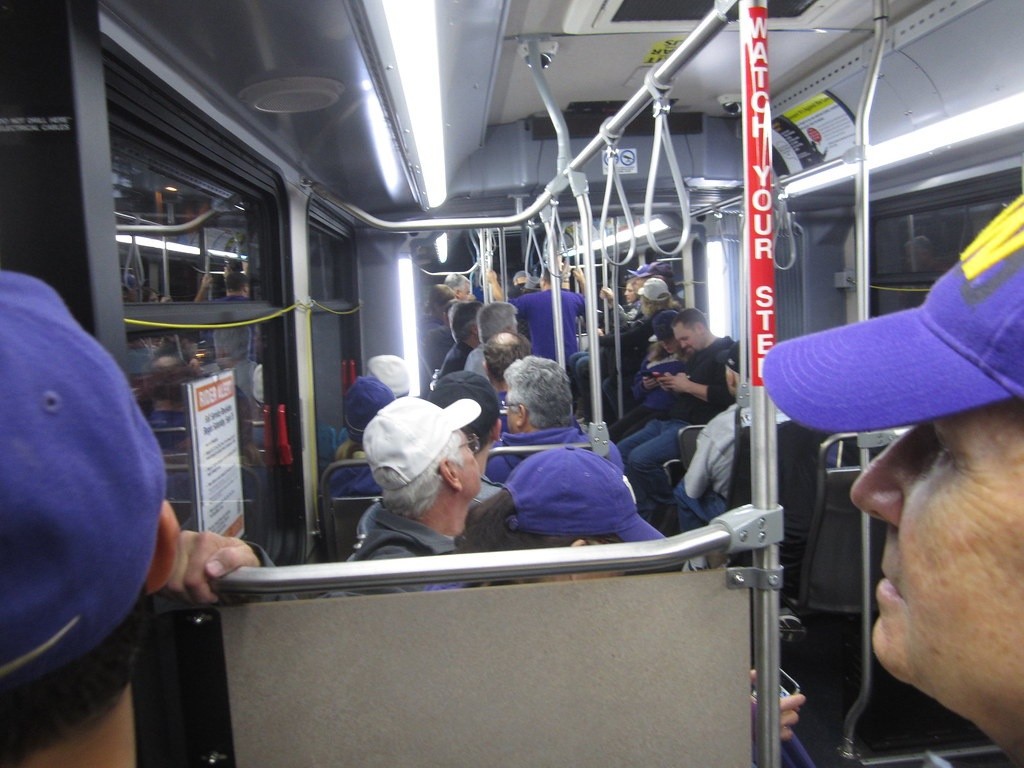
[[501, 400, 518, 409]]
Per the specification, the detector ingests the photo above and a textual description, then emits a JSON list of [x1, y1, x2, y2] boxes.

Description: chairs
[[321, 459, 383, 562], [201, 463, 262, 542], [780, 426, 913, 616], [153, 427, 193, 531]]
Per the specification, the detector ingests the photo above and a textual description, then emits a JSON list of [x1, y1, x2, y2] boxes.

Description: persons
[[762, 192, 1023, 768], [163, 254, 755, 610], [0, 270, 177, 768]]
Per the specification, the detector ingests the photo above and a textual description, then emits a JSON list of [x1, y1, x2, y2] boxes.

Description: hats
[[762, 193, 1024, 433], [344, 375, 394, 441], [367, 355, 409, 396], [524, 276, 541, 290], [501, 446, 666, 542], [627, 264, 650, 276], [0, 270, 166, 687], [637, 262, 674, 277], [653, 310, 679, 341], [725, 338, 748, 378], [362, 396, 482, 491], [513, 271, 531, 280], [427, 370, 499, 438], [637, 278, 671, 301]]
